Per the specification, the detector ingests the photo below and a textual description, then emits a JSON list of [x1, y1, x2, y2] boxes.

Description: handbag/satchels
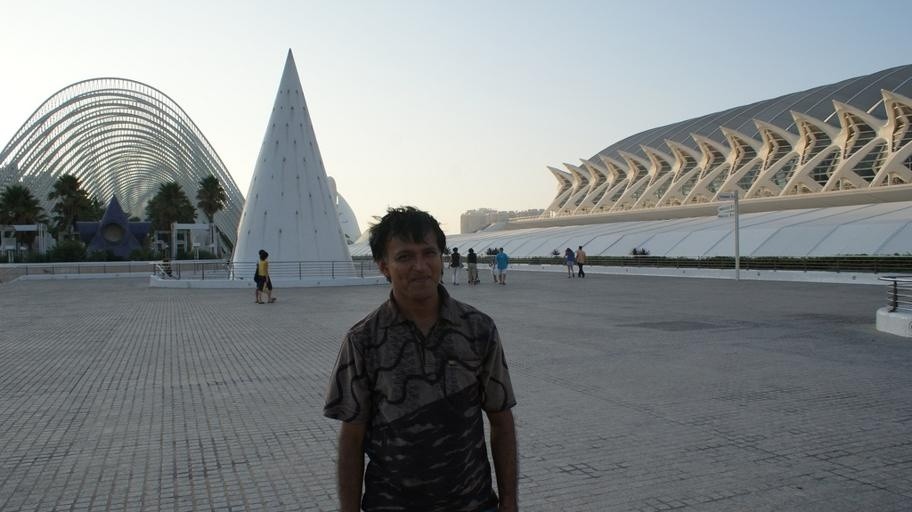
[[262, 282, 271, 294]]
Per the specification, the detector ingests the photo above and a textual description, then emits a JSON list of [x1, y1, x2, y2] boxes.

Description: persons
[[254, 249, 276, 304], [441, 248, 478, 286], [322, 207, 518, 512], [489, 248, 508, 284], [565, 246, 585, 278]]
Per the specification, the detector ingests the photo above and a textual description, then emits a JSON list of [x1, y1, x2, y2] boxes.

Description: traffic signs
[[718, 211, 736, 217], [716, 192, 736, 200], [718, 203, 735, 211]]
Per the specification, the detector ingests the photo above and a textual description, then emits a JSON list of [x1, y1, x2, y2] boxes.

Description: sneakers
[[267, 298, 276, 303], [568, 275, 585, 279], [440, 279, 506, 286], [254, 300, 264, 304]]
